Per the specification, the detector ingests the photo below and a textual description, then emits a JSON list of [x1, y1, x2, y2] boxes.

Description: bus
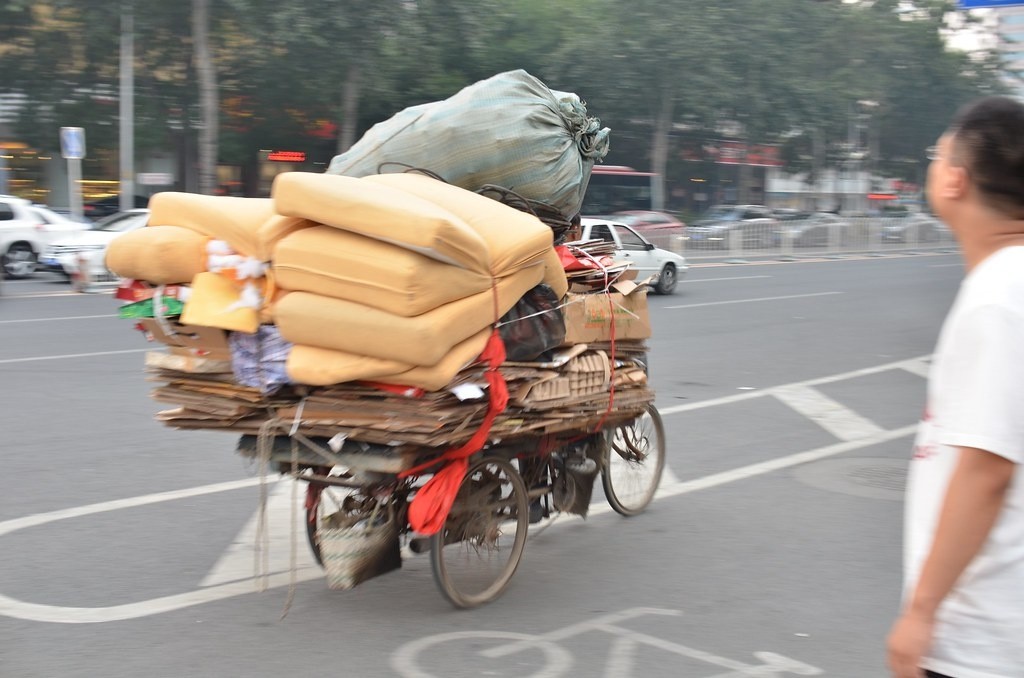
[[577, 165, 662, 221]]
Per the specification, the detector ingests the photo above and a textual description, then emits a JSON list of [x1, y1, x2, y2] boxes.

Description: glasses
[[924, 143, 951, 161]]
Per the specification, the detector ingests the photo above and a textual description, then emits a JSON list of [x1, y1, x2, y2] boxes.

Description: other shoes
[[565, 455, 597, 474], [540, 503, 557, 515]]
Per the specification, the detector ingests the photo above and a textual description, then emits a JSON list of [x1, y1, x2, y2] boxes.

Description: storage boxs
[[561, 290, 652, 345]]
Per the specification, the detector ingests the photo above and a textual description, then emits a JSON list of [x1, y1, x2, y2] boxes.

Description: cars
[[577, 217, 686, 298], [31, 205, 90, 237], [603, 211, 685, 250], [771, 212, 856, 247], [876, 210, 942, 243], [37, 208, 151, 282], [83, 194, 150, 217]]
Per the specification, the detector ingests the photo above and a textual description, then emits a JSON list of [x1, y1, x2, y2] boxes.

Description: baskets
[[317, 495, 403, 590]]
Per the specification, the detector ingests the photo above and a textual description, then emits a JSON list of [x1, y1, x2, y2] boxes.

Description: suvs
[[1, 194, 45, 278], [683, 204, 778, 250]]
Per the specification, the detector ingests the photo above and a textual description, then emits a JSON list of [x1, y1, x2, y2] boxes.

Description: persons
[[515, 214, 597, 523], [881, 96, 1024, 678]]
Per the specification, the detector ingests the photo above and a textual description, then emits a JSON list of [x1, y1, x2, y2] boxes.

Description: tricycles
[[236, 403, 667, 609]]
